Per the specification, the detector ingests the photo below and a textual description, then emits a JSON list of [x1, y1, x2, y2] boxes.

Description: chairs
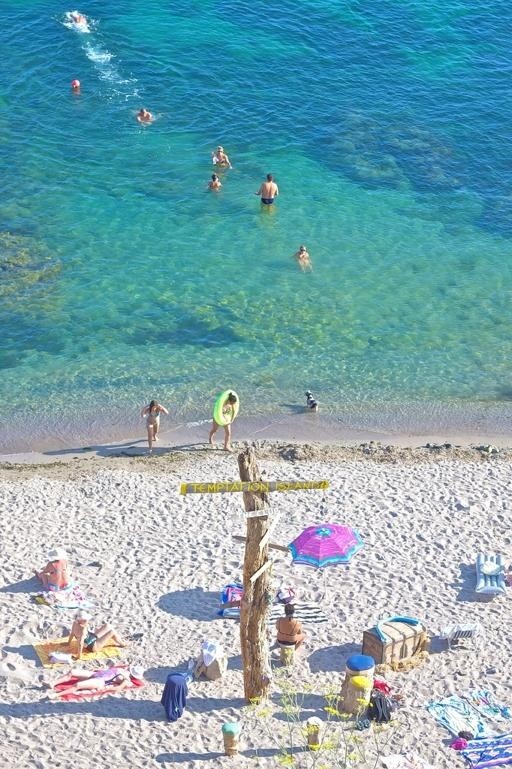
[[472, 552, 505, 595], [439, 622, 478, 653]]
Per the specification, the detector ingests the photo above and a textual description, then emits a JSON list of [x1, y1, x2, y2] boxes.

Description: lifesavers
[[214, 390, 240, 426]]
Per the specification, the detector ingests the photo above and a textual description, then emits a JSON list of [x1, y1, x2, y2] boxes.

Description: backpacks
[[372, 698, 391, 722]]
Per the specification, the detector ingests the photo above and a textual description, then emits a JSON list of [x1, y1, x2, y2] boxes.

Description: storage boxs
[[362, 618, 427, 671]]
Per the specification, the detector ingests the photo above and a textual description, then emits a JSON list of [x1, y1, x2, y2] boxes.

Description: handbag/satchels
[[450, 737, 466, 750], [458, 732, 473, 740]]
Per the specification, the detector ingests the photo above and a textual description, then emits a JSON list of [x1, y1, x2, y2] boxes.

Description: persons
[[34, 548, 143, 701], [71, 79, 80, 94], [142, 400, 169, 450], [439, 702, 478, 742], [275, 602, 304, 652], [210, 145, 279, 214], [71, 13, 86, 22], [209, 392, 237, 451], [138, 107, 153, 124], [296, 246, 313, 271]]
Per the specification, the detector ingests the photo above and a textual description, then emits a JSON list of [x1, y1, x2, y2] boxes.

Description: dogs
[[304, 390, 319, 412]]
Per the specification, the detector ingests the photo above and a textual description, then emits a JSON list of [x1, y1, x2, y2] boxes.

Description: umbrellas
[[289, 522, 358, 572]]
